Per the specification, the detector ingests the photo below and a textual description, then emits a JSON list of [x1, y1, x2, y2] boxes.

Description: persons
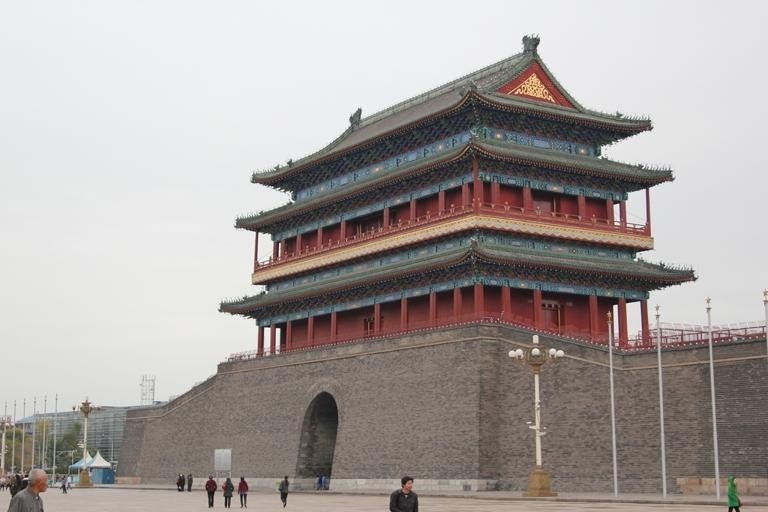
[[180, 473, 185, 490], [236, 476, 248, 508], [0, 468, 73, 512], [725, 475, 741, 512], [221, 478, 234, 508], [186, 472, 193, 492], [314, 474, 320, 489], [204, 475, 217, 508], [175, 473, 181, 490], [321, 474, 327, 489], [389, 476, 417, 511], [316, 473, 324, 489], [278, 475, 289, 507]]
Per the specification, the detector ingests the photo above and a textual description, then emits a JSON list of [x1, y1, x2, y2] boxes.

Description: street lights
[[0, 414, 15, 477], [508, 334, 565, 497], [72, 394, 101, 488]]
